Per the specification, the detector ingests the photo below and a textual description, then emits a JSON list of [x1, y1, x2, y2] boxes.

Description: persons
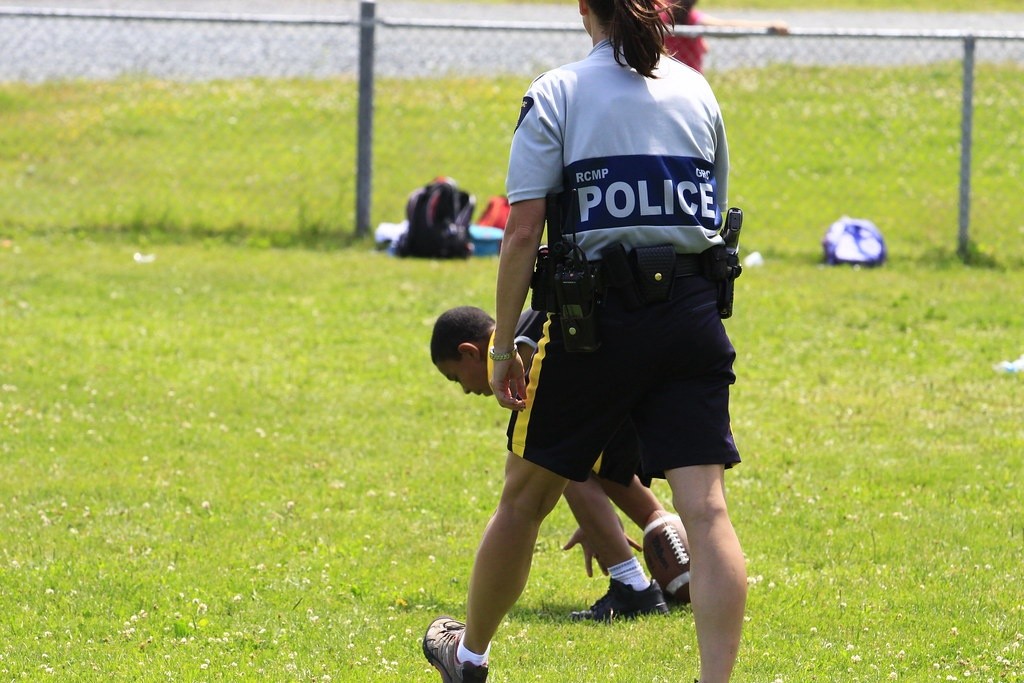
[[419, 1, 749, 682], [654, 0, 791, 75], [429, 304, 676, 623]]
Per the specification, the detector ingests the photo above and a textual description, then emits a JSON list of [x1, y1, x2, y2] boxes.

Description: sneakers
[[423, 615, 489, 683], [569, 577, 670, 622]]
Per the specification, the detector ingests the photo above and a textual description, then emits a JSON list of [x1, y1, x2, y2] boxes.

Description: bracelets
[[490, 342, 518, 360]]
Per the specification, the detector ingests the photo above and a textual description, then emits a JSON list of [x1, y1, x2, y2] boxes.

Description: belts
[[593, 253, 705, 289]]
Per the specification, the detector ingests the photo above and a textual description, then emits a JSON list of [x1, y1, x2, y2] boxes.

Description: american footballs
[[644, 508, 690, 602]]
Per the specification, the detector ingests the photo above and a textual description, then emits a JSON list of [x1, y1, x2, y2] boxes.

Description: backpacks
[[477, 195, 512, 252], [824, 215, 888, 268], [396, 178, 475, 258]]
